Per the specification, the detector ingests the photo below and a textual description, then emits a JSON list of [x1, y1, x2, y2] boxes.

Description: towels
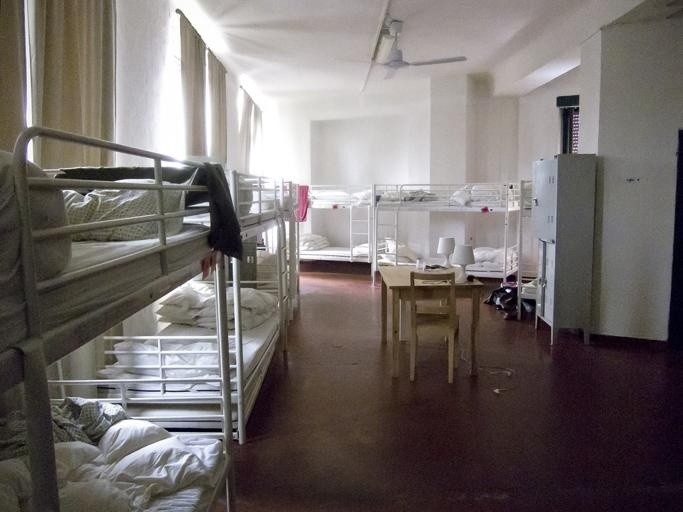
[[297, 186, 309, 222]]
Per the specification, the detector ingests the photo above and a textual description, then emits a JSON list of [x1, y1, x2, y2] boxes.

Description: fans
[[335, 20, 466, 81]]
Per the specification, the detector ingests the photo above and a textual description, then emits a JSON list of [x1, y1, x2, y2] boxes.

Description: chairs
[[411, 272, 461, 383]]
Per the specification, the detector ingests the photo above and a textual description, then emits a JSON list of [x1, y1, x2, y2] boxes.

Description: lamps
[[437, 237, 455, 269], [452, 244, 475, 282]]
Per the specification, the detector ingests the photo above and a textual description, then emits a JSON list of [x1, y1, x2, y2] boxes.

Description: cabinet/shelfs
[[534, 154, 596, 345]]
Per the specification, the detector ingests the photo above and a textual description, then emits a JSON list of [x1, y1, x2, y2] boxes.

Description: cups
[[416, 258, 424, 269]]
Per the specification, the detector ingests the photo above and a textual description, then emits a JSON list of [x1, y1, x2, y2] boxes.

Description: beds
[[302, 184, 372, 277], [267, 181, 310, 321], [0, 124, 233, 511], [84, 173, 289, 444], [373, 181, 517, 287], [516, 179, 537, 321]]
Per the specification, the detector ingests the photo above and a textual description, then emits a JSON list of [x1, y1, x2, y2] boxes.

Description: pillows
[[353, 242, 381, 256], [350, 189, 370, 200], [114, 339, 235, 379], [384, 236, 418, 262], [381, 189, 410, 201], [379, 253, 410, 265], [54, 168, 200, 240]]
